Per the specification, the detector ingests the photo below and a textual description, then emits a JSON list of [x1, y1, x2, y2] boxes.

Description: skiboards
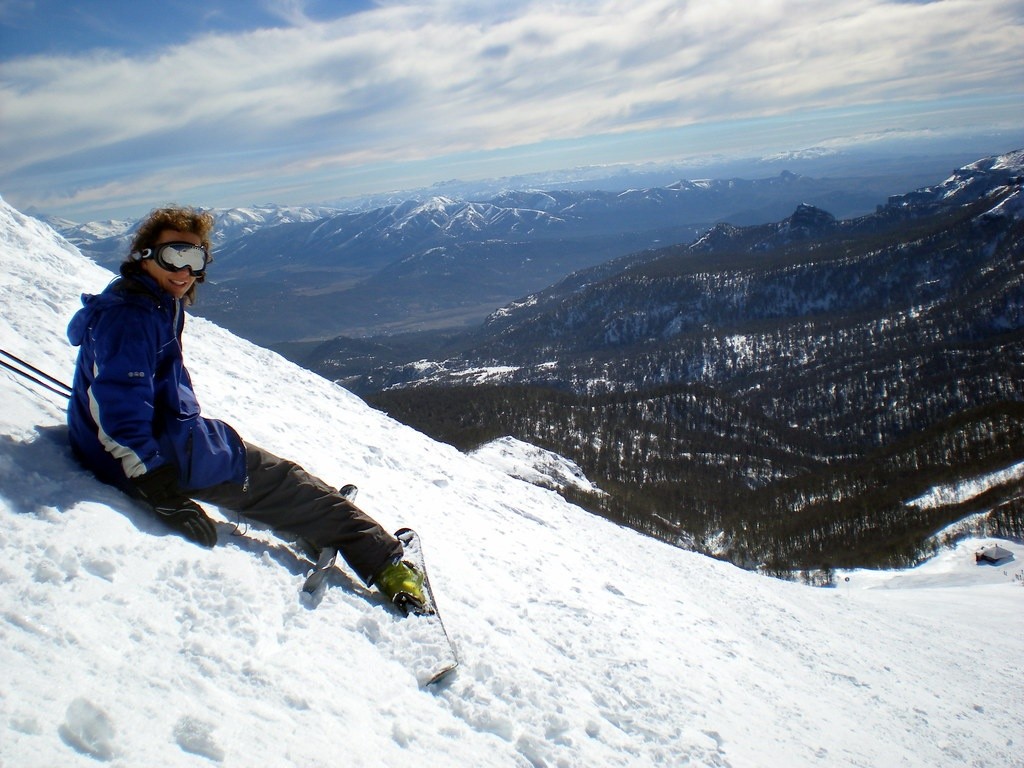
[[303, 484, 359, 594], [394, 527, 459, 685]]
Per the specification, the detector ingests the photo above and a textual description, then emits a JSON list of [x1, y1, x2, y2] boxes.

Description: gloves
[[151, 492, 218, 548]]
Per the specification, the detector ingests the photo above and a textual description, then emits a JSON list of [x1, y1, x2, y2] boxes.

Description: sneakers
[[375, 559, 426, 608]]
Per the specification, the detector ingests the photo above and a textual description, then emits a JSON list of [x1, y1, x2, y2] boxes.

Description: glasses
[[131, 240, 208, 278]]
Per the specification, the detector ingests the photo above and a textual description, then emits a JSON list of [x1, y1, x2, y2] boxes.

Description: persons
[[66, 203, 427, 618]]
